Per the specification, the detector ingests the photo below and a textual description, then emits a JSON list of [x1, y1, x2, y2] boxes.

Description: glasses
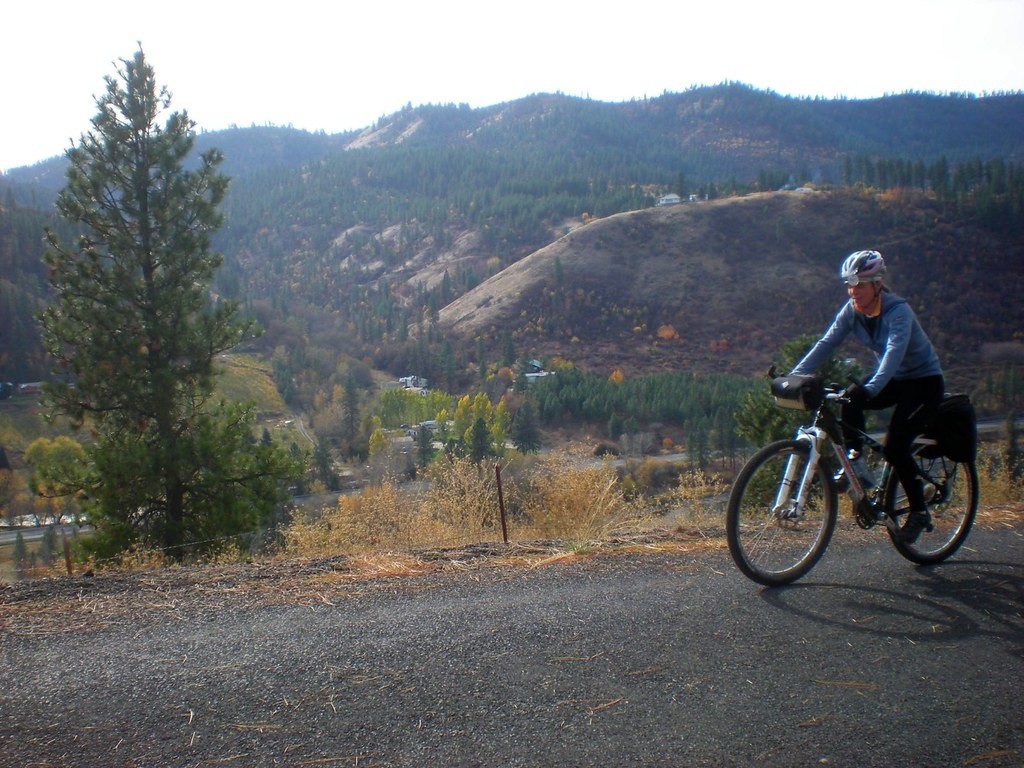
[[843, 275, 874, 286]]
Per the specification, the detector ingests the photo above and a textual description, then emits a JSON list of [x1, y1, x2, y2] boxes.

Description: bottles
[[848, 449, 875, 488]]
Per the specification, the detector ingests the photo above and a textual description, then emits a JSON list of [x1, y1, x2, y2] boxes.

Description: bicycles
[[724, 363, 980, 584]]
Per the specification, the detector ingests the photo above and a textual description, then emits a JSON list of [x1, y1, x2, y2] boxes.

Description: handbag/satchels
[[912, 392, 976, 463]]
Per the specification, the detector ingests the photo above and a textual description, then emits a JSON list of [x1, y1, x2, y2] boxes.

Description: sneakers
[[830, 469, 856, 492], [900, 511, 931, 544]]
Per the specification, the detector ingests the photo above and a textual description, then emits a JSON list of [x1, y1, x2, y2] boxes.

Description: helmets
[[839, 250, 886, 279]]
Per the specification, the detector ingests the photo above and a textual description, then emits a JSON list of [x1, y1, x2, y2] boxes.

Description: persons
[[785, 250, 945, 544]]
